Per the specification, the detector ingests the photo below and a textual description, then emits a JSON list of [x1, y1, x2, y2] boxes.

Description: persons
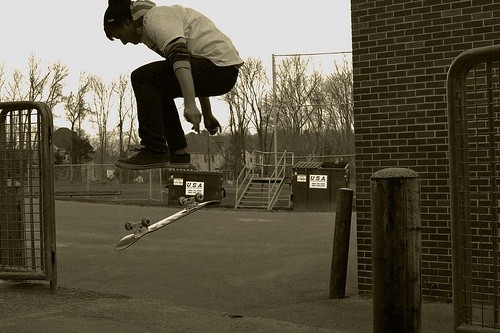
[[104, 0, 245, 169]]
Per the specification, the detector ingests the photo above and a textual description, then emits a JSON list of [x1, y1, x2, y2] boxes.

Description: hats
[[104, 0, 131, 26]]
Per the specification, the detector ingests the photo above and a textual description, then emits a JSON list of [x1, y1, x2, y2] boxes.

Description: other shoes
[[168, 153, 191, 168], [115, 148, 170, 169]]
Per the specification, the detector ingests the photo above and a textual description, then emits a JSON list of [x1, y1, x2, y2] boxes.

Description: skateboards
[[114, 192, 220, 251]]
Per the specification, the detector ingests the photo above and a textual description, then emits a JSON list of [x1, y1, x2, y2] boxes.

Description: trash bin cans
[[291, 161, 350, 213], [167, 167, 224, 208]]
